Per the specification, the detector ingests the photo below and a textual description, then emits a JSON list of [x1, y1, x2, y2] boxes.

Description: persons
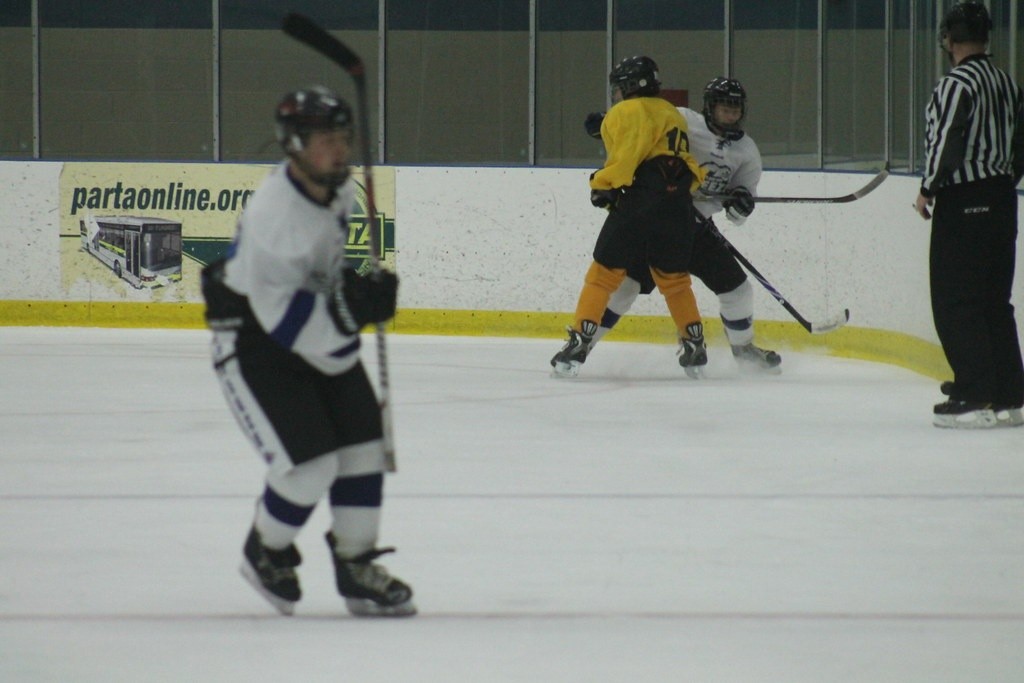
[[917, 2, 1024, 432], [550, 56, 783, 381], [201, 85, 420, 619]]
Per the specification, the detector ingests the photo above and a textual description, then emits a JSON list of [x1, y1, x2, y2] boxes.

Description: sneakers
[[239, 525, 301, 615], [326, 531, 416, 617], [551, 319, 598, 378], [724, 328, 782, 375], [932, 397, 1024, 430], [679, 321, 708, 380]]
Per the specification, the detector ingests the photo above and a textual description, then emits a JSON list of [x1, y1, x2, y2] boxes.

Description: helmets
[[940, 0, 992, 30], [609, 56, 662, 101], [274, 85, 356, 134], [704, 77, 748, 141]]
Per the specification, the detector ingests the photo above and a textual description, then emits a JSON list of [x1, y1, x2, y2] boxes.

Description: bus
[[78, 216, 183, 289]]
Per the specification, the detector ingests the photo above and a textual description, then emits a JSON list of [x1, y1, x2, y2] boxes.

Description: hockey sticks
[[280, 15, 396, 472], [697, 161, 891, 204], [691, 204, 852, 336]]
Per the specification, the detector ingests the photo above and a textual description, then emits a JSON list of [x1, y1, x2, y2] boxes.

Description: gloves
[[584, 112, 607, 140], [326, 267, 398, 336], [723, 186, 755, 225], [591, 190, 609, 208]]
